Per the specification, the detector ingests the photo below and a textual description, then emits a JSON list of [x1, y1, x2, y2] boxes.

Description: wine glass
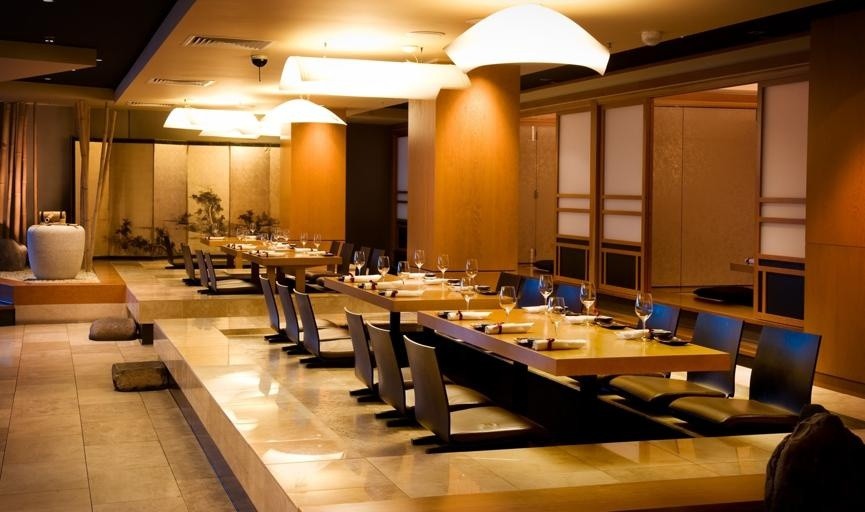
[[377, 256, 390, 293], [498, 285, 517, 324], [459, 277, 475, 313], [397, 261, 410, 290], [437, 254, 449, 284], [413, 248, 425, 280], [634, 291, 653, 342], [465, 256, 478, 295], [545, 297, 566, 340], [353, 251, 366, 285], [249, 223, 257, 235], [538, 274, 554, 317], [578, 281, 597, 329], [313, 233, 322, 257], [300, 231, 309, 255]]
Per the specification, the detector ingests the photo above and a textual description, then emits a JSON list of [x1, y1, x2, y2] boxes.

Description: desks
[[200, 237, 342, 293]]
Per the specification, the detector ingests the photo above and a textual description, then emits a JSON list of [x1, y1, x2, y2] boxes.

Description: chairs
[[306, 240, 386, 290], [162, 236, 257, 293]]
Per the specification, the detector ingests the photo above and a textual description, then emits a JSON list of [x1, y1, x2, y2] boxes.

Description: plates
[[596, 321, 630, 329], [653, 335, 692, 346]]
